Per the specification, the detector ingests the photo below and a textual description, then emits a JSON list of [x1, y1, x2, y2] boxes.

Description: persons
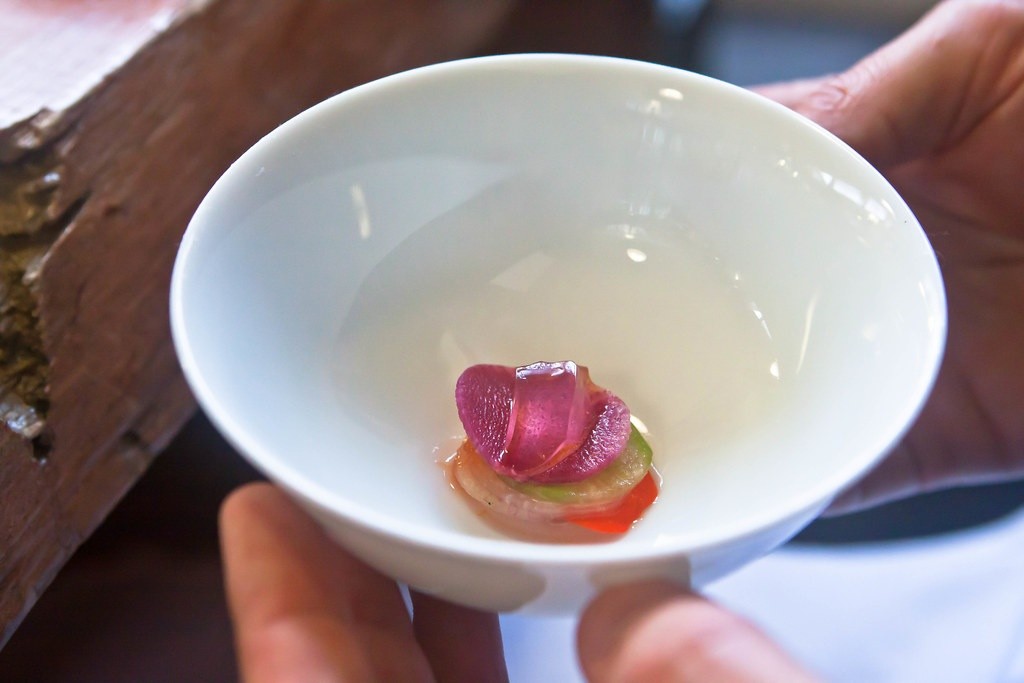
[[217, 1, 1024, 681]]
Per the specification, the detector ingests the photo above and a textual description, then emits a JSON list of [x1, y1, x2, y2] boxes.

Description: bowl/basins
[[169, 53, 947, 611]]
